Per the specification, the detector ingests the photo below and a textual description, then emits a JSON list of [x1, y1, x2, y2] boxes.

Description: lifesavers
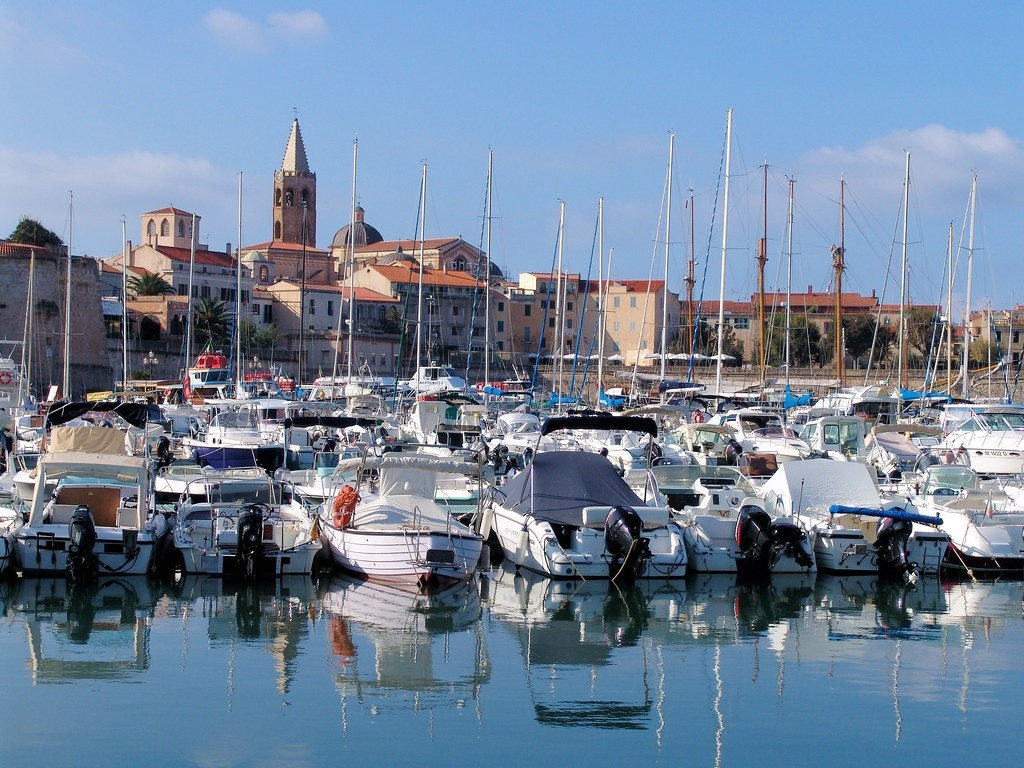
[[476, 381, 484, 389], [331, 485, 356, 527], [0, 371, 13, 387], [693, 411, 704, 423]]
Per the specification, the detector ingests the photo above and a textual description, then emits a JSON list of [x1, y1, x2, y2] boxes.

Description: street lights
[[248, 356, 262, 375], [143, 351, 158, 380]]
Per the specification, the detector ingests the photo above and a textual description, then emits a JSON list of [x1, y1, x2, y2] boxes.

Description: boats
[[174, 480, 323, 573], [483, 409, 687, 578], [878, 461, 1023, 580], [17, 452, 170, 574], [317, 441, 484, 583], [757, 459, 951, 574], [651, 465, 818, 571]]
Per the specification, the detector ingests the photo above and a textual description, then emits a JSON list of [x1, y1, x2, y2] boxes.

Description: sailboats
[[0, 110, 1024, 475]]
